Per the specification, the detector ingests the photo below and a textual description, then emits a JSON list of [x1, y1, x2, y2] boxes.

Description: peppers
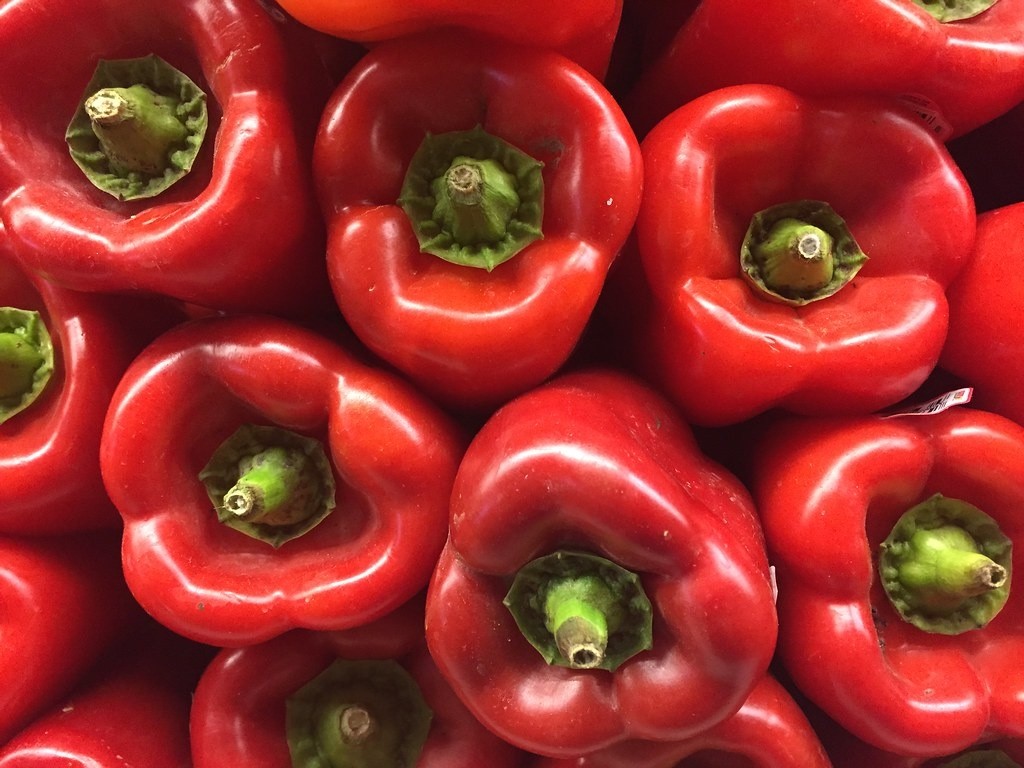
[[0, 0, 1024, 768]]
